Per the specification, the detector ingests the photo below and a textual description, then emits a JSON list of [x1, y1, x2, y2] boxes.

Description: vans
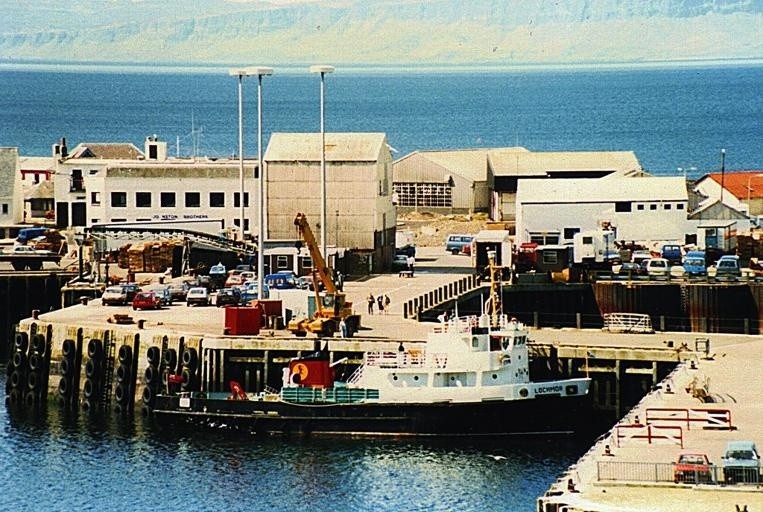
[[444, 233, 474, 257], [600, 242, 762, 284]]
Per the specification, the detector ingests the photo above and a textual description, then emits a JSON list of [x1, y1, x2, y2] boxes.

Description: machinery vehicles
[[283, 209, 362, 338]]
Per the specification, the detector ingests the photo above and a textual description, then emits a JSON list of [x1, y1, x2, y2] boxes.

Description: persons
[[383, 293, 391, 315], [365, 291, 375, 314], [336, 270, 343, 293], [377, 293, 384, 314], [337, 317, 346, 338], [408, 255, 415, 277]]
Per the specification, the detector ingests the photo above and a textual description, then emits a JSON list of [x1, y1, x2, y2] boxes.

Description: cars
[[461, 244, 470, 257], [95, 262, 348, 314], [668, 452, 714, 485], [1, 244, 54, 255], [394, 245, 416, 258]]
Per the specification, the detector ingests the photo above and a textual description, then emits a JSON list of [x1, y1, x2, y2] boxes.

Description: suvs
[[719, 440, 761, 484]]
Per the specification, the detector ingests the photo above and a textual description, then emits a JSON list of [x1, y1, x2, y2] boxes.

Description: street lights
[[229, 62, 249, 247], [673, 166, 699, 179], [746, 173, 762, 216], [720, 148, 725, 203], [311, 63, 336, 261], [245, 65, 277, 300]]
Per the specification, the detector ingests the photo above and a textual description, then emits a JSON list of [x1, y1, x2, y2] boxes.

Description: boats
[[139, 250, 601, 444]]
